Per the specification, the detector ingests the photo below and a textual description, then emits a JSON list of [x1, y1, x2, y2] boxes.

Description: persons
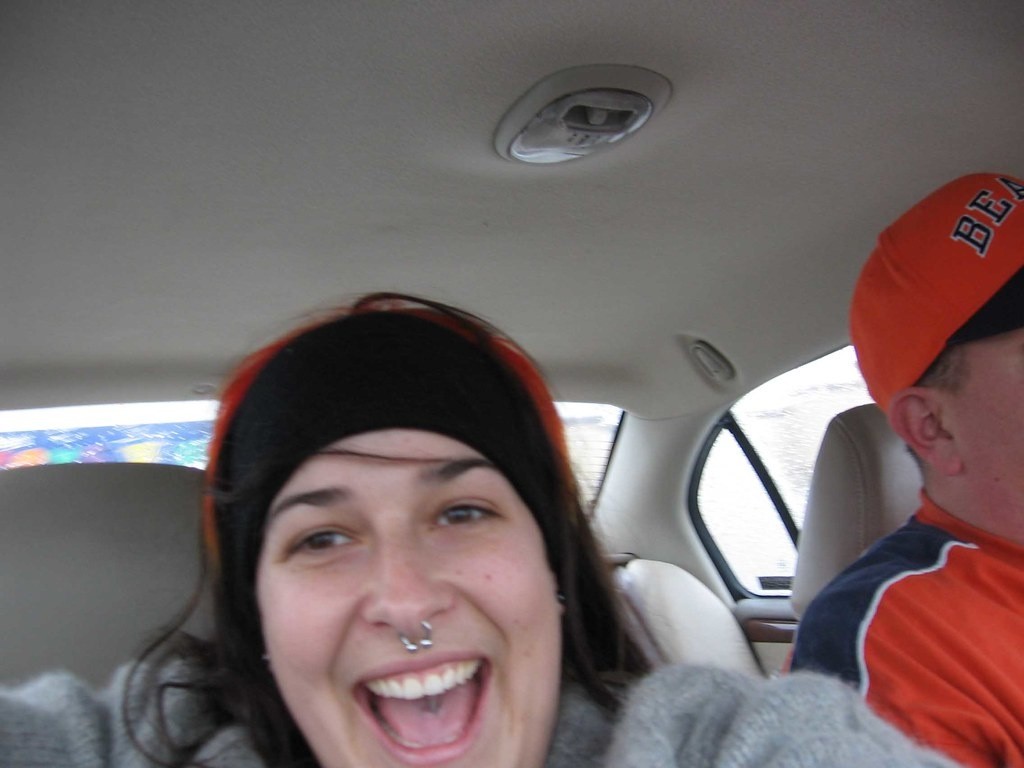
[[781, 171, 1024, 768], [0, 293, 964, 768]]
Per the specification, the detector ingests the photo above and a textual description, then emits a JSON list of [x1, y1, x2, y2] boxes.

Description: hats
[[848, 173, 1024, 412]]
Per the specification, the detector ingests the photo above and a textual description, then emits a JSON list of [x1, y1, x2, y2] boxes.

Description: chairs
[[0, 461, 214, 692]]
